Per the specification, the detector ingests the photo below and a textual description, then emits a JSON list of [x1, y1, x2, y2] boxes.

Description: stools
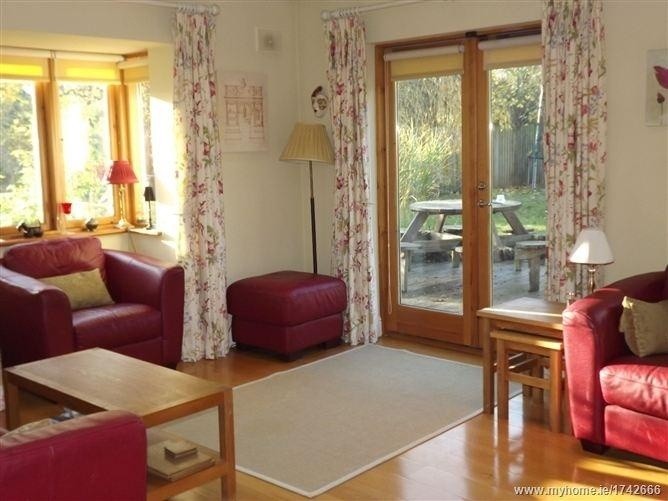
[[225, 271, 347, 362], [489, 330, 564, 434]]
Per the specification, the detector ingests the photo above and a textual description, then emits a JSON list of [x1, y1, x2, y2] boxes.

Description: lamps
[[567, 228, 615, 295], [107, 160, 139, 230], [277, 123, 336, 274]]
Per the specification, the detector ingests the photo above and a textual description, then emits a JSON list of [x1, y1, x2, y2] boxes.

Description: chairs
[[560, 265, 668, 465], [0, 236, 185, 373]]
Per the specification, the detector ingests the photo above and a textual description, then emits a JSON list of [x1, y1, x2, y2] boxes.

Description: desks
[[475, 297, 568, 415], [400, 199, 531, 244]]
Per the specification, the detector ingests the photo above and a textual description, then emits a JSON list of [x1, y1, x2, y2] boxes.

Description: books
[[146, 436, 214, 482]]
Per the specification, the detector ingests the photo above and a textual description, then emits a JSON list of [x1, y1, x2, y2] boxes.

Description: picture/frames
[[644, 49, 668, 128]]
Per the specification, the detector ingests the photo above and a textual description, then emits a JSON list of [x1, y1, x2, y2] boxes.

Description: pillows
[[618, 296, 668, 358], [35, 267, 115, 312]]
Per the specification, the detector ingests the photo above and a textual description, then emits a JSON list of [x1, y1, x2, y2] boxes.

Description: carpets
[[154, 344, 523, 499]]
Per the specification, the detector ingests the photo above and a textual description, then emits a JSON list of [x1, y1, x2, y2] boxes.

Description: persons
[[309, 85, 330, 118]]
